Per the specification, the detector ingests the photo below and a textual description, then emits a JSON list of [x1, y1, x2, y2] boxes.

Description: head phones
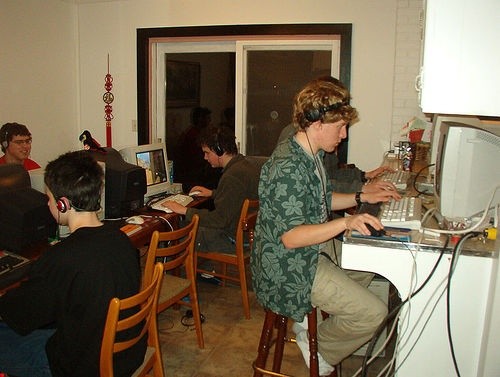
[[181, 310, 205, 326], [1, 124, 12, 148], [302, 100, 350, 122], [215, 125, 223, 157]]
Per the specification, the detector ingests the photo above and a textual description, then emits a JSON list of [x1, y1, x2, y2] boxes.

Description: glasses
[[10, 138, 32, 144]]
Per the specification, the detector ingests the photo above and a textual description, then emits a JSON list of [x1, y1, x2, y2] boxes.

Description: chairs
[[100, 199, 263, 377]]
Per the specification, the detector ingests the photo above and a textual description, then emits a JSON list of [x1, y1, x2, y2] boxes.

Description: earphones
[[50, 165, 65, 213]]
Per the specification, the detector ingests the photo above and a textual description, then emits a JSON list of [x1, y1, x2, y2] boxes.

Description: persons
[[250, 75, 404, 377], [160, 124, 258, 284], [0, 151, 148, 377], [0, 122, 41, 171], [172, 108, 237, 190]]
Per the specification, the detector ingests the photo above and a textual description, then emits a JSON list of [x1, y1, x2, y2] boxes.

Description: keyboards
[[377, 170, 408, 191], [380, 196, 422, 230], [149, 194, 194, 214], [0, 254, 31, 279]]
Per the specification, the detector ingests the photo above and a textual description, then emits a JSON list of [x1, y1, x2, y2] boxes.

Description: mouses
[[188, 191, 201, 197], [364, 223, 385, 235], [124, 216, 145, 225]]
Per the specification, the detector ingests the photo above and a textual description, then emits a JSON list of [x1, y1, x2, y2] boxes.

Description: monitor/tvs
[[87, 147, 148, 218], [433, 120, 500, 225], [426, 113, 500, 177], [0, 163, 55, 253], [119, 142, 170, 205]]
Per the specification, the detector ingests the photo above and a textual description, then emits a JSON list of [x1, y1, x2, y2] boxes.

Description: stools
[[251, 304, 337, 377]]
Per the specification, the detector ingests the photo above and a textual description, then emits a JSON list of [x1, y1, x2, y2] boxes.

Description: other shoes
[[197, 273, 221, 287]]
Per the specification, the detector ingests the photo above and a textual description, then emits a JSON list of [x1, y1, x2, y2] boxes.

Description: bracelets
[[361, 170, 368, 183], [355, 191, 363, 205]]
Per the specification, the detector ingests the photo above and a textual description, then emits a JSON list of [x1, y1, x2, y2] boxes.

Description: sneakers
[[295, 329, 335, 376], [292, 316, 309, 335]]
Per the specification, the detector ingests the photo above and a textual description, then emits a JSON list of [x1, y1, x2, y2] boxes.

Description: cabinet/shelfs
[[414, 0, 500, 117]]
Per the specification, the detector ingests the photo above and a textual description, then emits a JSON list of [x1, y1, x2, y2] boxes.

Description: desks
[[0, 193, 208, 290], [340, 160, 495, 377]]
